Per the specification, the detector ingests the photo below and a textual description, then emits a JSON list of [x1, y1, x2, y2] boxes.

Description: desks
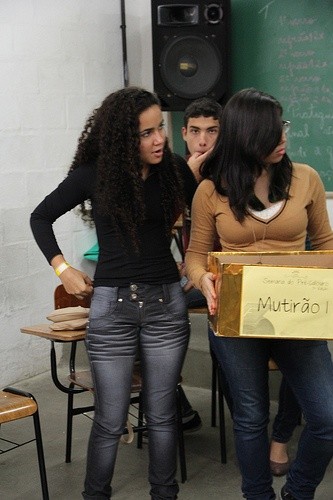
[[19, 322, 91, 393]]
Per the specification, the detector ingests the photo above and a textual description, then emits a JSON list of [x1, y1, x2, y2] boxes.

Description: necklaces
[[240, 190, 274, 265]]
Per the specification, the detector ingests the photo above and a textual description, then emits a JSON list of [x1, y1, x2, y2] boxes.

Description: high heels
[[270, 442, 291, 476]]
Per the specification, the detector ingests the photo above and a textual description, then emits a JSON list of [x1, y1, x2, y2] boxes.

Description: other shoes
[[281, 479, 299, 500], [141, 410, 203, 439]]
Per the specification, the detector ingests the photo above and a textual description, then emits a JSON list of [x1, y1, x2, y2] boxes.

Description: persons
[[147, 98, 224, 435], [183, 88, 332, 499]]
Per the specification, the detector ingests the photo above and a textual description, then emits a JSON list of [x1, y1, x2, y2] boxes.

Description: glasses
[[282, 120, 291, 133]]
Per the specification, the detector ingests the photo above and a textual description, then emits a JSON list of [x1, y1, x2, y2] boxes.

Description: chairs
[[54, 283, 281, 484], [0, 387, 49, 500]]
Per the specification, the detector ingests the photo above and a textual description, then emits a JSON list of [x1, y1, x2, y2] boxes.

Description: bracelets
[[51, 261, 72, 277], [29, 87, 199, 500]]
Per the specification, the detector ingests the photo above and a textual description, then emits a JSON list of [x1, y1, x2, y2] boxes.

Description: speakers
[[151, 0, 230, 111]]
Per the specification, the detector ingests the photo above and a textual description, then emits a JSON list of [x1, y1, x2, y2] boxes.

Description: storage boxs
[[207, 251, 333, 339]]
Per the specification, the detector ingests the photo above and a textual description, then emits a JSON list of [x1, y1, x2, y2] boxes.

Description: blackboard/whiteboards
[[168, 0, 333, 200]]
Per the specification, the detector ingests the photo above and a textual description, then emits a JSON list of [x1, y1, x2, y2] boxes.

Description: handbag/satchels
[[47, 307, 89, 331]]
